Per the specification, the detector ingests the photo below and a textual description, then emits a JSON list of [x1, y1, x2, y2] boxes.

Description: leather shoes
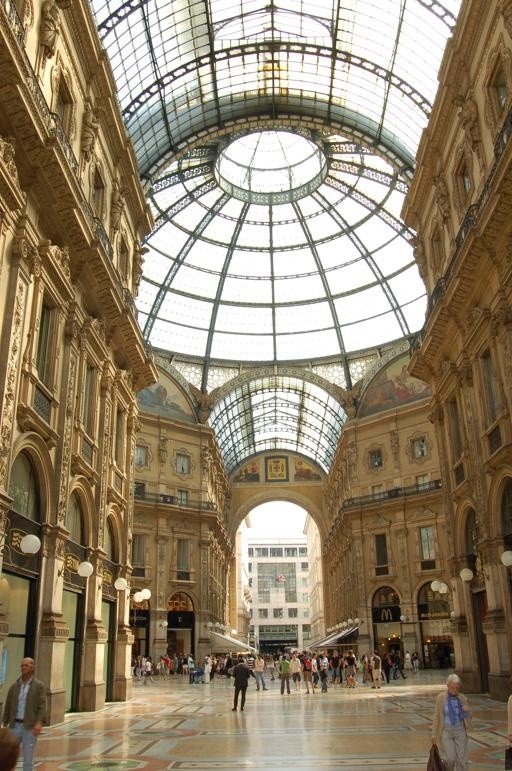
[[241, 708, 245, 711], [232, 707, 237, 711]]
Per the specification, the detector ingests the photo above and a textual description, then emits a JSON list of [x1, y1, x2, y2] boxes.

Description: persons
[[112, 187, 129, 234], [429, 178, 448, 217], [507, 695, 512, 744], [450, 95, 480, 148], [239, 460, 306, 481], [131, 649, 422, 695], [399, 362, 427, 395], [40, 0, 61, 57], [0, 726, 20, 771], [81, 106, 106, 163], [1, 657, 49, 771], [430, 672, 473, 770], [133, 245, 149, 287], [231, 658, 250, 711]]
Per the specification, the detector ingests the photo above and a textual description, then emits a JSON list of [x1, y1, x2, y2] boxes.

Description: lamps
[[160, 621, 168, 632], [326, 618, 364, 633], [400, 615, 409, 622], [431, 567, 489, 618], [206, 621, 232, 635], [0, 516, 41, 568], [500, 550, 512, 566], [57, 553, 93, 578], [96, 566, 151, 603]]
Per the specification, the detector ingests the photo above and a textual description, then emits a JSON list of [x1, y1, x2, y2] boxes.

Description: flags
[[275, 574, 286, 584]]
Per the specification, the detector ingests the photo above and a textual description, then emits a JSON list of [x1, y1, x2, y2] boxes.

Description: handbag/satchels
[[427, 744, 443, 770], [505, 747, 512, 771]]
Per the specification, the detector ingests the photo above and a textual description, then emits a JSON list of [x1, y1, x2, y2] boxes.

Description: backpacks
[[304, 660, 311, 670]]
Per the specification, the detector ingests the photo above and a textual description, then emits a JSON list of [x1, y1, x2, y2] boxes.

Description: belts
[[15, 719, 23, 723]]
